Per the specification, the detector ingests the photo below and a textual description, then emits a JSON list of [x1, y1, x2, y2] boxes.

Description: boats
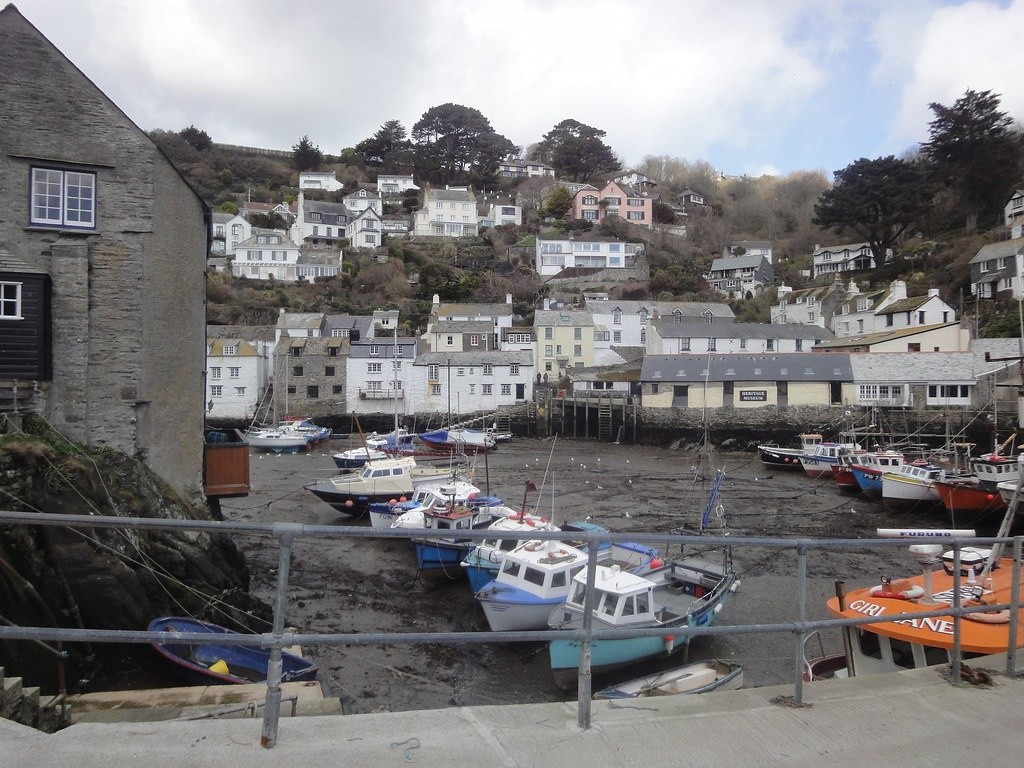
[[367, 436, 518, 531], [546, 337, 741, 692], [475, 471, 664, 631], [460, 431, 612, 591], [332, 445, 388, 475], [147, 616, 316, 684], [409, 435, 518, 571], [758, 364, 1024, 683]]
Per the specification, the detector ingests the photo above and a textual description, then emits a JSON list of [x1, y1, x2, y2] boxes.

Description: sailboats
[[302, 328, 473, 516], [418, 358, 496, 452], [233, 349, 333, 452]]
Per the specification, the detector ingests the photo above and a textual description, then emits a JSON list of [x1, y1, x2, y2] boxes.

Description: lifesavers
[[525, 541, 545, 551], [951, 597, 1012, 623], [913, 459, 929, 466], [433, 504, 451, 514], [509, 511, 531, 520], [869, 580, 924, 599], [548, 548, 569, 559], [526, 515, 548, 527]]
